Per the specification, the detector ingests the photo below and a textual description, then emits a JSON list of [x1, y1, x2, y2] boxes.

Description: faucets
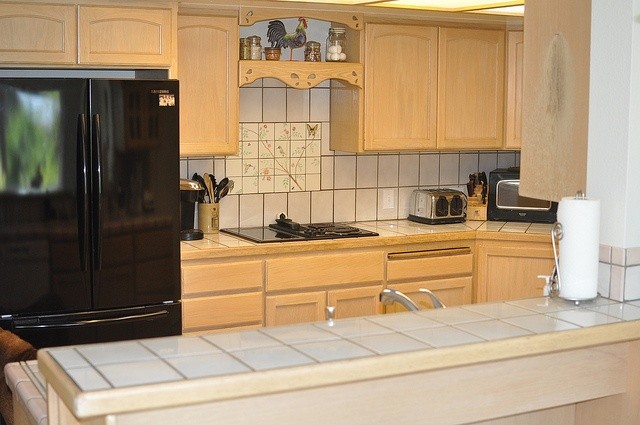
[[380, 288, 421, 311]]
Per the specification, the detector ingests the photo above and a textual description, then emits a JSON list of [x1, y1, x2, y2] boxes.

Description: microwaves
[[488, 166, 557, 224]]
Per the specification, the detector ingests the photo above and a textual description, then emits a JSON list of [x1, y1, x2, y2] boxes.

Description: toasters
[[409, 188, 468, 224]]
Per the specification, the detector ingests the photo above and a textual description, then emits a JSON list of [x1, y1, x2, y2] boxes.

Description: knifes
[[467, 171, 488, 203]]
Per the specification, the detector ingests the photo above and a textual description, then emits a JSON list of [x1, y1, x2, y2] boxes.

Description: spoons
[[215, 177, 229, 204], [195, 174, 211, 203], [218, 186, 229, 202], [203, 172, 216, 204]]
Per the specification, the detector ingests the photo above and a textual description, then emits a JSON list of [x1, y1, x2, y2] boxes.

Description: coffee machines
[[180, 177, 205, 241]]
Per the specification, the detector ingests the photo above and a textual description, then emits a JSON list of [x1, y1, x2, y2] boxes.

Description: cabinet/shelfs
[[178, 14, 239, 157], [238, 7, 363, 90], [385, 248, 473, 313], [518, 0, 592, 203], [265, 251, 386, 328], [0, 1, 179, 75], [329, 21, 522, 152], [180, 260, 265, 334], [477, 244, 559, 304]]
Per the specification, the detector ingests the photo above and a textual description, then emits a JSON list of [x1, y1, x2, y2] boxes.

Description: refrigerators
[[0, 77, 181, 349]]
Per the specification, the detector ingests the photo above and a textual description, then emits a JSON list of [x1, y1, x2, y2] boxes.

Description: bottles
[[304, 41, 322, 62], [250, 36, 263, 60], [326, 26, 348, 61], [266, 48, 280, 60], [240, 36, 252, 59]]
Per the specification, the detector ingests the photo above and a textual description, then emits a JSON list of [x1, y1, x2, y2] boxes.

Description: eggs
[[325, 43, 346, 63]]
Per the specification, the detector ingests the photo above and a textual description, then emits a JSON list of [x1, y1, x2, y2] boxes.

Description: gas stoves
[[280, 213, 365, 237]]
